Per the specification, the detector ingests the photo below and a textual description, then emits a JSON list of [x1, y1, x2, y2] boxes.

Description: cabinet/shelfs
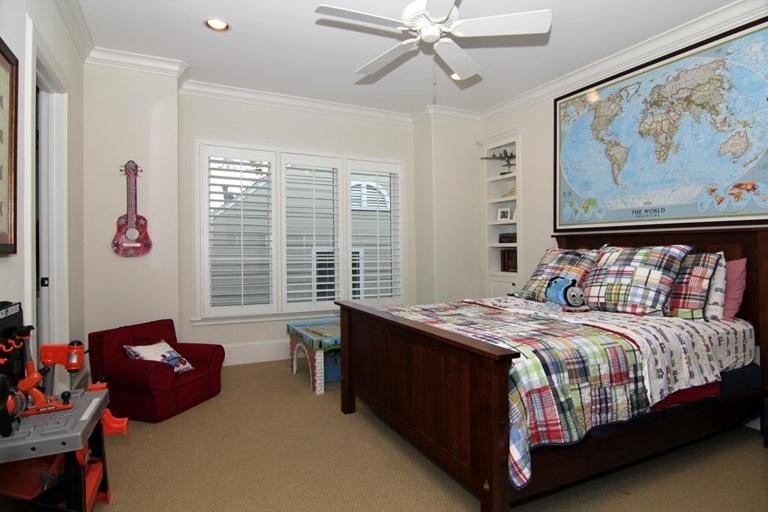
[[485, 139, 521, 297]]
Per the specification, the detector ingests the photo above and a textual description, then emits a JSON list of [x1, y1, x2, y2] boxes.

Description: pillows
[[670, 253, 721, 320], [702, 250, 726, 321], [513, 246, 605, 302], [579, 244, 693, 316], [724, 258, 746, 320], [123, 340, 195, 376]]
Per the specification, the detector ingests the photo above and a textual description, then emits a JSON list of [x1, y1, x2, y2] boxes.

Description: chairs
[[88, 319, 225, 424]]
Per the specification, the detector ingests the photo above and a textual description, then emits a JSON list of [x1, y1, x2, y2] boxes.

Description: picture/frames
[[0, 36, 17, 255], [552, 15, 767, 232], [498, 208, 511, 220]]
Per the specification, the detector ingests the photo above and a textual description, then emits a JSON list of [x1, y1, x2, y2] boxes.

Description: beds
[[334, 225, 767, 512]]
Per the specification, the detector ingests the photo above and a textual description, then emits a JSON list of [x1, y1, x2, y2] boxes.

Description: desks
[[287, 319, 341, 395]]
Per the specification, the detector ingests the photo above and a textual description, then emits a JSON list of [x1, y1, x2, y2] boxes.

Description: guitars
[[112, 161, 152, 256]]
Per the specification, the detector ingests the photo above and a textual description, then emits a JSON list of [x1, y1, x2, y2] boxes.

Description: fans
[[314, 0, 552, 86]]
